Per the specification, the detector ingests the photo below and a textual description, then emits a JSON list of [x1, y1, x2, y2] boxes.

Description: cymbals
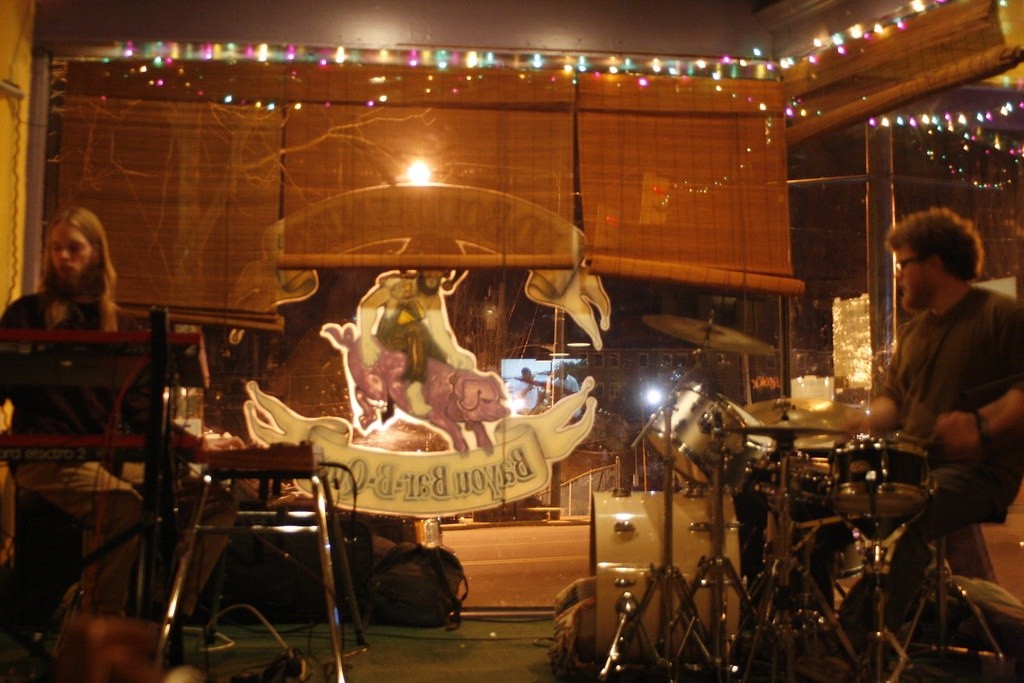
[[742, 399, 866, 449], [641, 313, 775, 354], [730, 420, 848, 440]]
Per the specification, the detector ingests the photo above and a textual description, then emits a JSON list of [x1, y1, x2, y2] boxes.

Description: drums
[[589, 489, 742, 671], [831, 442, 929, 515], [717, 391, 776, 484], [741, 463, 833, 503], [644, 381, 750, 486], [743, 494, 862, 585]]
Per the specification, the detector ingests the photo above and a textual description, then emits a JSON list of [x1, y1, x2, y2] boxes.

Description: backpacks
[[359, 542, 469, 632]]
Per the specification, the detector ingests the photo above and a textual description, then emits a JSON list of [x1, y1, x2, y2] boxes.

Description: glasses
[[894, 254, 935, 272]]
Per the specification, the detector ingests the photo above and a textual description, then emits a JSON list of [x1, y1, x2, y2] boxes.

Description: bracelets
[[966, 409, 994, 449]]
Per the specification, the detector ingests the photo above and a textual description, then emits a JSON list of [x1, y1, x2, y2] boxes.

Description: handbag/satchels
[[177, 462, 371, 626]]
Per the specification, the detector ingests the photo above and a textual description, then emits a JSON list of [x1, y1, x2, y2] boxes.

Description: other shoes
[[169, 618, 184, 668]]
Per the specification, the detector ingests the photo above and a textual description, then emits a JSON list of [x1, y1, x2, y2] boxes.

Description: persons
[[0, 207, 240, 666], [512, 367, 547, 415], [548, 363, 581, 417], [776, 206, 1024, 683]]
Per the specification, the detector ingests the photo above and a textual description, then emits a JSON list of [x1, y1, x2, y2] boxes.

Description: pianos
[[0, 430, 200, 464], [0, 327, 211, 392], [197, 440, 325, 472]]
